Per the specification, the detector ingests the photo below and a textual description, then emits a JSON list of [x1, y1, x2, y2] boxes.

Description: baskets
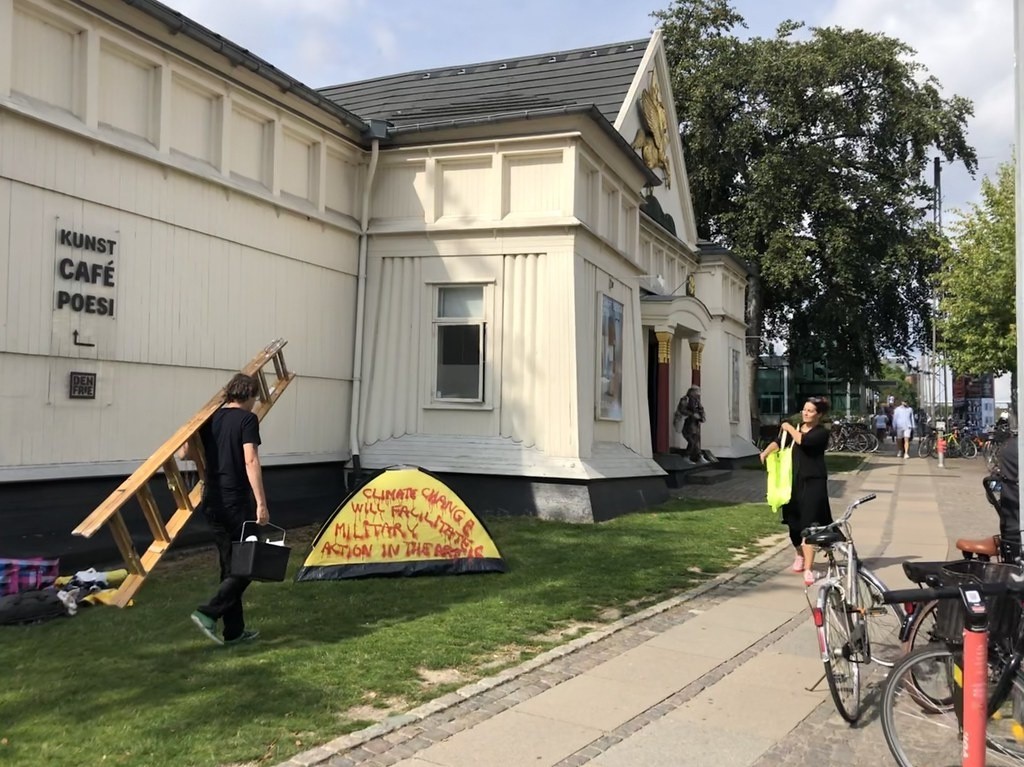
[[993, 530, 1024, 566], [230, 520, 292, 582], [934, 560, 1024, 643]]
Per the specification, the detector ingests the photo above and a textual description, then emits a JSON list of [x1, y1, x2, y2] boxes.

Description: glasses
[[808, 397, 821, 403]]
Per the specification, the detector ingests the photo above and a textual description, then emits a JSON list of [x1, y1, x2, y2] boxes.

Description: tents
[[295, 463, 511, 582]]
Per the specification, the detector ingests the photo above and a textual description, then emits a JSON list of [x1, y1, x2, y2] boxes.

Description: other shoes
[[793, 555, 804, 572], [904, 454, 909, 458], [698, 454, 710, 463], [684, 455, 696, 465], [804, 569, 815, 584], [897, 450, 903, 457]]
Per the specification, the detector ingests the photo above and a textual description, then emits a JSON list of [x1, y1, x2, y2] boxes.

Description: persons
[[178, 373, 270, 646], [759, 397, 831, 585], [892, 399, 927, 458], [677, 384, 710, 465], [1000, 409, 1008, 419], [872, 408, 893, 443]]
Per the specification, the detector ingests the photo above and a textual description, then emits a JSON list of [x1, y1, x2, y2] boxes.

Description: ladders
[[70, 336, 297, 610]]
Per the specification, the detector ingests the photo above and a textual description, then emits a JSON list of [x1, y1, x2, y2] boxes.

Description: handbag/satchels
[[0, 558, 59, 595], [765, 422, 799, 513], [673, 397, 689, 433], [0, 588, 64, 625]]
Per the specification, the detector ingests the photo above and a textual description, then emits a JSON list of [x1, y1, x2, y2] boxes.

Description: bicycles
[[800, 494, 906, 723], [918, 418, 980, 458], [828, 416, 878, 454], [879, 533, 1024, 766]]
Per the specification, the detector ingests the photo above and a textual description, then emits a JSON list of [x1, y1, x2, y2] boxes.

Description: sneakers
[[224, 630, 259, 643], [191, 609, 224, 646]]
